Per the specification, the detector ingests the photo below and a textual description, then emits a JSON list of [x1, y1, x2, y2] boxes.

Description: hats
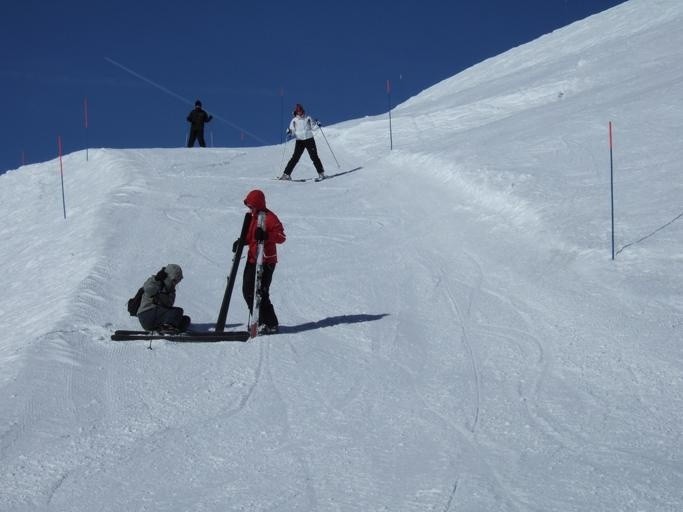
[[195, 99, 202, 108], [296, 105, 303, 113]]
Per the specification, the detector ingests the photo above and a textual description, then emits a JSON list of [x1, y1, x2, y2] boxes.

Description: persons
[[279, 103, 324, 179], [232, 189, 286, 329], [186, 101, 213, 147], [137, 264, 190, 335]]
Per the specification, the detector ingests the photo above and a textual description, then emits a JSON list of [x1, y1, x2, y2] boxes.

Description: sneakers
[[279, 174, 290, 180], [319, 174, 325, 179], [247, 312, 279, 335], [158, 324, 179, 335]]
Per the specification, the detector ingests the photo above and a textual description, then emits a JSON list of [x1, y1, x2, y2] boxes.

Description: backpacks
[[127, 287, 145, 316]]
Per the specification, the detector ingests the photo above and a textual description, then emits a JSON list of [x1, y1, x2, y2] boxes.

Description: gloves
[[254, 227, 269, 239], [287, 128, 291, 136], [232, 237, 248, 253], [316, 120, 321, 125], [155, 266, 169, 281]]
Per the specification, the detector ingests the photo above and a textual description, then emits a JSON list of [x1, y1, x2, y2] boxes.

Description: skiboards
[[110, 330, 249, 341], [215, 211, 267, 338], [271, 175, 328, 182]]
